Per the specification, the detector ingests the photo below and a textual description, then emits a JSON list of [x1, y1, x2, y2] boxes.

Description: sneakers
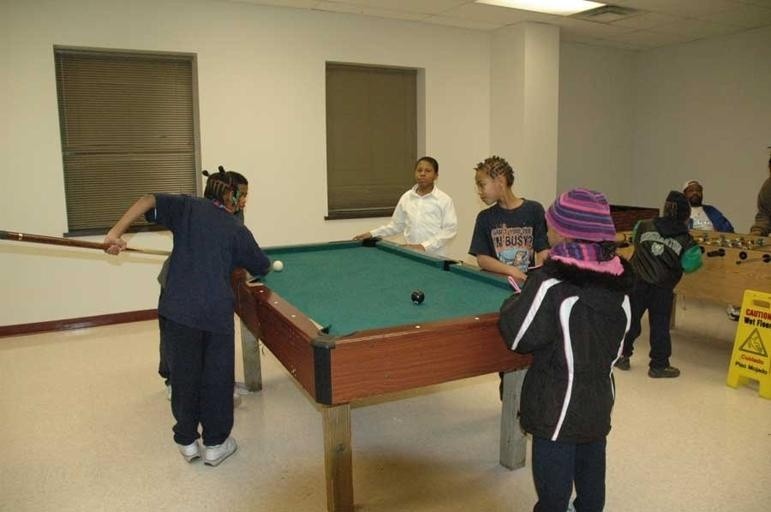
[[203, 437, 237, 467], [647, 365, 679, 379], [614, 356, 631, 371], [178, 440, 201, 463]]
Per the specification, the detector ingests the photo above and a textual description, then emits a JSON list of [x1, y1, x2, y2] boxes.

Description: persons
[[750, 157, 771, 236], [156, 170, 249, 408], [101, 165, 272, 468], [682, 179, 734, 233], [468, 156, 553, 282], [615, 189, 704, 379], [497, 186, 642, 511], [352, 155, 460, 256]]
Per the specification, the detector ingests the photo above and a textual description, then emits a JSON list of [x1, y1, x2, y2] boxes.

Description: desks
[[231, 235, 535, 511], [608, 200, 660, 231]]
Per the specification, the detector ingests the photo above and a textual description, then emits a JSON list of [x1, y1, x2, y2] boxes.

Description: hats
[[683, 179, 703, 192], [545, 187, 616, 242]]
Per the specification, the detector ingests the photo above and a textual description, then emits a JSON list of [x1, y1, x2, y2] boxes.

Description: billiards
[[273, 260, 283, 272], [412, 291, 425, 303]]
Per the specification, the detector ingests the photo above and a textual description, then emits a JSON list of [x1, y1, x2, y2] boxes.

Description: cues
[[0, 231, 172, 255]]
